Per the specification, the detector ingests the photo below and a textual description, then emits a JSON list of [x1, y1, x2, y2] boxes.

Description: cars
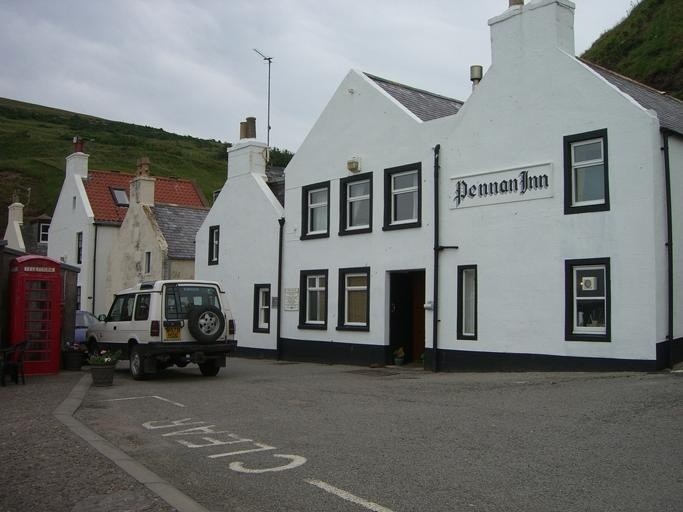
[[76, 309, 108, 346]]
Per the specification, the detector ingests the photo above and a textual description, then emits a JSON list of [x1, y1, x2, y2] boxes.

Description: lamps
[[347, 158, 361, 173]]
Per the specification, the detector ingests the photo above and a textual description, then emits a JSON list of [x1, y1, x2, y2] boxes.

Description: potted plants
[[85, 350, 123, 387]]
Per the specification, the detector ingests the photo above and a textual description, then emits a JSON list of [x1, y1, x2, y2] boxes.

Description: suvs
[[86, 280, 238, 381]]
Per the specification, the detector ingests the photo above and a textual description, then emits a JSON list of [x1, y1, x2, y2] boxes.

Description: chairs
[[130, 301, 189, 319], [0, 339, 30, 386]]
